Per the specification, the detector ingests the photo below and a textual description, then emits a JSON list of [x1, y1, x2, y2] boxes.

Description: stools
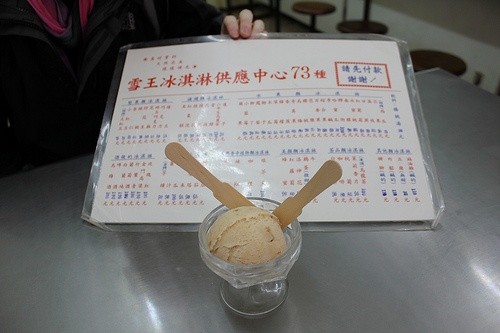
[[410, 49, 468, 77], [336, 20, 389, 37], [290, 1, 336, 31]]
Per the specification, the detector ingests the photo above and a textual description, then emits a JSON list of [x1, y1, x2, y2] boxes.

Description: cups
[[197, 196, 301, 315]]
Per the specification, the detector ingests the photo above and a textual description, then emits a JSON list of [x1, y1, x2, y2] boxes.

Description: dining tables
[[0, 68, 500, 333]]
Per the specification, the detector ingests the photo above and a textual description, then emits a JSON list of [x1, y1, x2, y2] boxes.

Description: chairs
[[222, 0, 283, 34]]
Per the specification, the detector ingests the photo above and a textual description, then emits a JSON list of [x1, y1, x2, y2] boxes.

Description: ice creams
[[205, 205, 287, 268]]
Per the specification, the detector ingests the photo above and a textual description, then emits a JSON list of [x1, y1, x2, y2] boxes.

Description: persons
[[0, 0, 270, 180]]
